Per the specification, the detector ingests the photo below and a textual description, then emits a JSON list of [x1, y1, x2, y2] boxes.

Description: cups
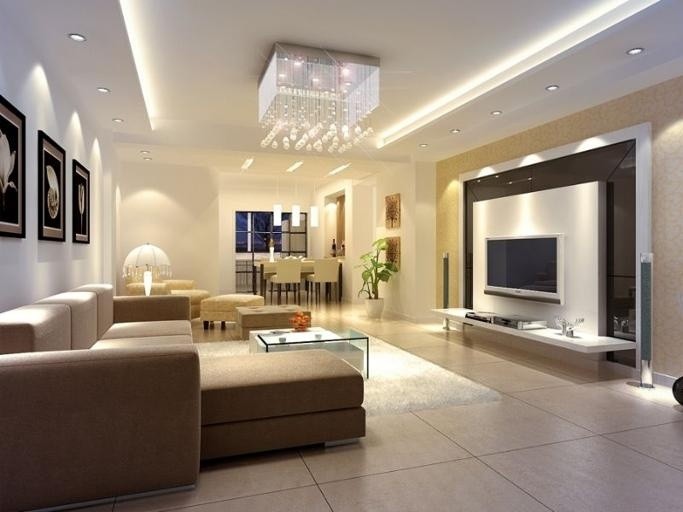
[[315, 333, 322, 339], [279, 336, 286, 342], [279, 254, 307, 261]]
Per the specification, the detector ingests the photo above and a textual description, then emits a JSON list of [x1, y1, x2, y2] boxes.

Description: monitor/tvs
[[484, 233, 565, 306]]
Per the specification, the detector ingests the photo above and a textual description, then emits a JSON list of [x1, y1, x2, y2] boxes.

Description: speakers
[[443, 251, 450, 330], [639, 253, 654, 388]]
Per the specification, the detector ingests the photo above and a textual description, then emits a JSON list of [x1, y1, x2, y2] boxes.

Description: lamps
[[272, 176, 318, 226], [256, 41, 380, 155], [121, 242, 173, 295]]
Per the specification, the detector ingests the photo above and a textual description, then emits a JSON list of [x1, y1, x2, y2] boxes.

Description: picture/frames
[[0, 93, 90, 244]]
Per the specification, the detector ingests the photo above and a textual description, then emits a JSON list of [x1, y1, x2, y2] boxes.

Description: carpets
[[192, 326, 503, 416]]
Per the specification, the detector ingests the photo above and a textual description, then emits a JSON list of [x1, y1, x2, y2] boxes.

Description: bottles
[[561, 322, 573, 337], [290, 309, 309, 331], [614, 316, 629, 332], [332, 238, 345, 257]]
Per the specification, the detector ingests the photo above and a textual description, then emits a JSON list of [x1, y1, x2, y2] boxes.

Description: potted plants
[[354, 238, 399, 319]]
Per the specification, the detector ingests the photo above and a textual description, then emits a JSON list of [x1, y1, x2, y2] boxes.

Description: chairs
[[251, 256, 344, 306], [121, 267, 209, 321]]
